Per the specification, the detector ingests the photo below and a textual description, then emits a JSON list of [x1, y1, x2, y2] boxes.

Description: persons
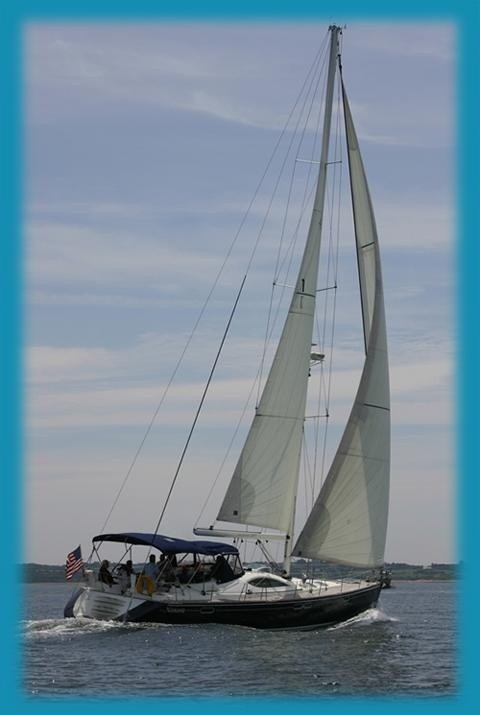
[[97, 552, 236, 592]]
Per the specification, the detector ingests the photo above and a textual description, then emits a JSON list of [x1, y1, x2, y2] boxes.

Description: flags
[[65, 545, 84, 582]]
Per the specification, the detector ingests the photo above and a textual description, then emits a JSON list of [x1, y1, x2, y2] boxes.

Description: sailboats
[[63, 23, 389, 632]]
